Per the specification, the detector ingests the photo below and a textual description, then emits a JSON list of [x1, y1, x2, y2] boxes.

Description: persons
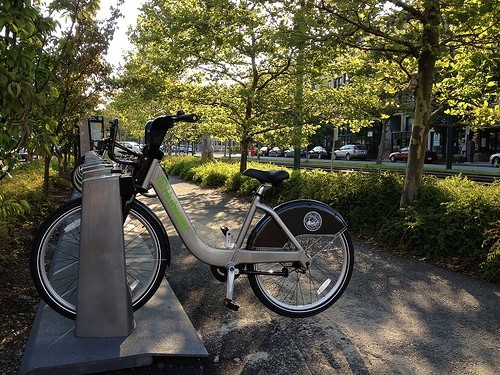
[[250, 144, 255, 157]]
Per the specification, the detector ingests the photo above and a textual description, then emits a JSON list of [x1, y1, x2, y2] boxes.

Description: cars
[[114, 142, 144, 155], [284, 146, 309, 157], [490, 151, 500, 167], [305, 145, 331, 160], [268, 146, 283, 157], [260, 146, 272, 156], [388, 147, 432, 163]]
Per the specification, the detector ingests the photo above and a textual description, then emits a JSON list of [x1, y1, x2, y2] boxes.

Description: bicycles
[[27, 108, 355, 323]]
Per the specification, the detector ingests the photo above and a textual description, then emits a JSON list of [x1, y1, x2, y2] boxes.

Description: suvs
[[332, 144, 369, 161]]
